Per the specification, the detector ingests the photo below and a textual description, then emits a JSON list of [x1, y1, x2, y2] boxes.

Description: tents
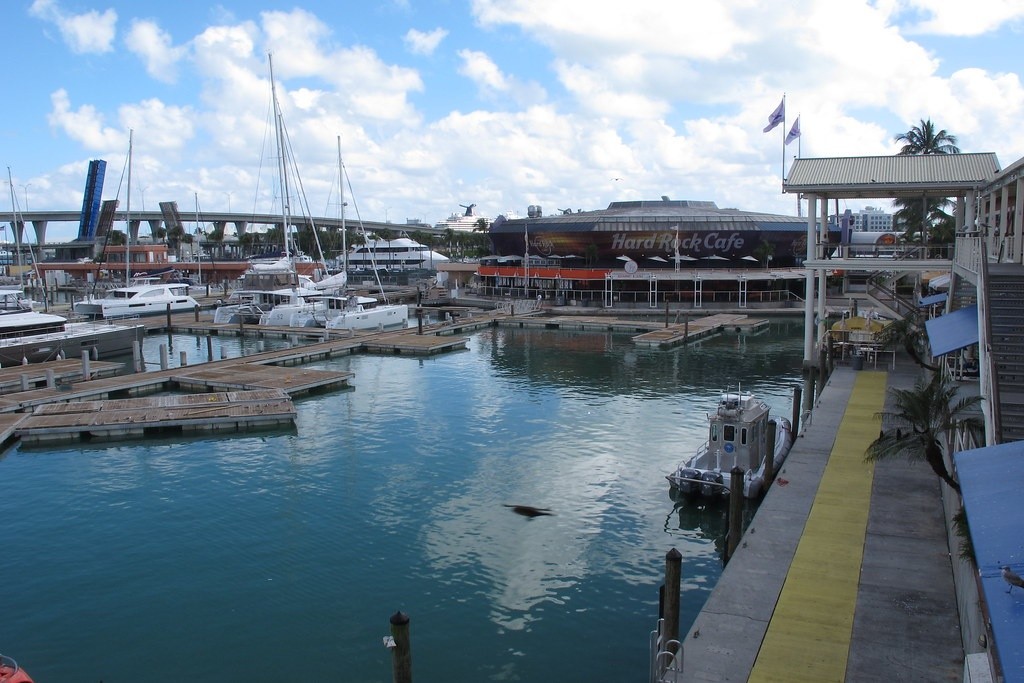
[[830, 314, 886, 362]]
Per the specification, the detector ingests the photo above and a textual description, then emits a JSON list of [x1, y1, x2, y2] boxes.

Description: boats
[[0, 167, 144, 368], [326, 230, 451, 272], [74, 127, 201, 318], [664, 382, 792, 498]]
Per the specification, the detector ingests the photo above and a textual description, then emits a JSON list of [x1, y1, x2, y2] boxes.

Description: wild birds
[[999, 566, 1024, 594]]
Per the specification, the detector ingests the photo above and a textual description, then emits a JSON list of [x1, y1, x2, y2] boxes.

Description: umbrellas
[[699, 254, 732, 269], [616, 254, 634, 268], [647, 255, 668, 268], [478, 253, 585, 267], [740, 255, 759, 268], [669, 255, 699, 269]]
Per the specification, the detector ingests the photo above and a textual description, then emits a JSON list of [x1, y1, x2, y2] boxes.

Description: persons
[[533, 291, 544, 310], [17, 297, 23, 306]]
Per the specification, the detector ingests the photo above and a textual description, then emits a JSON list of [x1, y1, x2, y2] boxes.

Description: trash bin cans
[[582, 298, 589, 306], [852, 354, 865, 371]]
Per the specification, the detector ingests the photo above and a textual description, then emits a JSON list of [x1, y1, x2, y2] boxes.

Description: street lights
[[19, 183, 32, 213]]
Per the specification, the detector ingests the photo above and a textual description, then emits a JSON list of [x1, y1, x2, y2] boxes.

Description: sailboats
[[214, 53, 408, 330]]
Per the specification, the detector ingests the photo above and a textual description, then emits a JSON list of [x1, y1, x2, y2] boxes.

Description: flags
[[762, 98, 785, 133], [784, 117, 800, 145]]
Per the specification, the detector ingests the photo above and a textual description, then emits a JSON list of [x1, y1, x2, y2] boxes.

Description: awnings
[[929, 273, 952, 291], [925, 304, 979, 358], [919, 292, 948, 305], [953, 441, 1024, 682]]
